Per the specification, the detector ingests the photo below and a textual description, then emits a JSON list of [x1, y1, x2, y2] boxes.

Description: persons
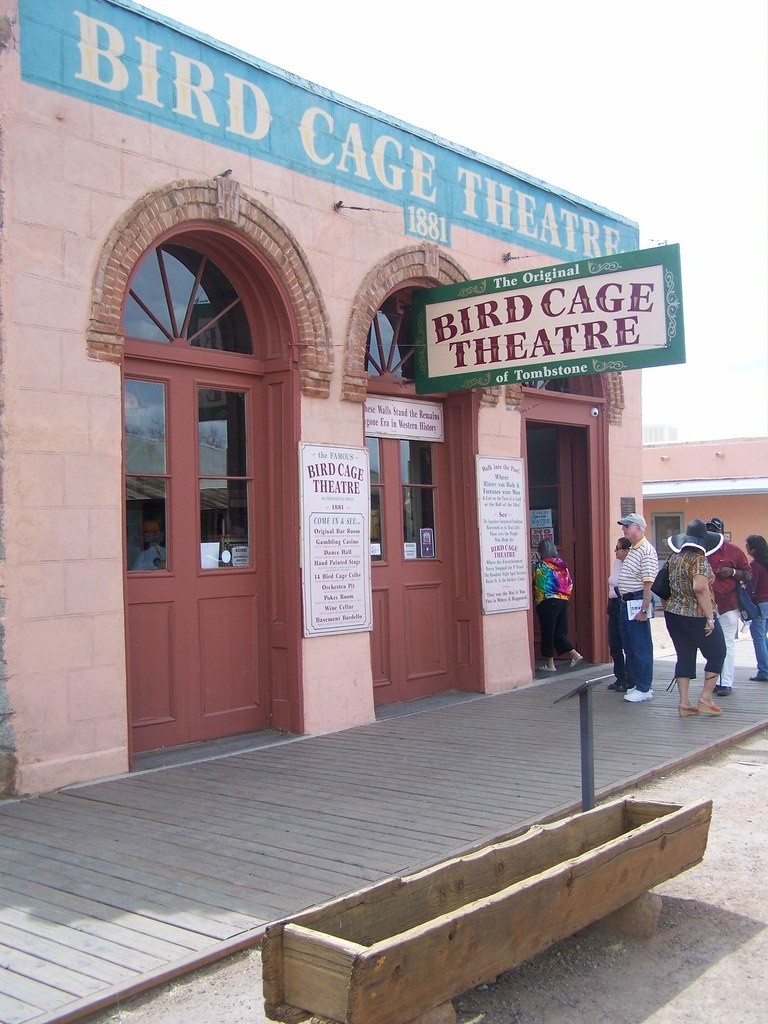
[[534, 539, 583, 672], [133, 521, 166, 570], [604, 513, 768, 717]]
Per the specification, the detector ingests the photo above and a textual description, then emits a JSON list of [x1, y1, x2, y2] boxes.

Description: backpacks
[[735, 569, 763, 621]]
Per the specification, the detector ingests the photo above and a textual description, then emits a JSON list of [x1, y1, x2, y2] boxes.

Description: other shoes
[[714, 685, 733, 697], [608, 680, 624, 690], [750, 676, 768, 682], [615, 680, 636, 692]]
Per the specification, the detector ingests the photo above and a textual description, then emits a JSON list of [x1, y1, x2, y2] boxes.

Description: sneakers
[[627, 684, 654, 694], [623, 690, 655, 703]]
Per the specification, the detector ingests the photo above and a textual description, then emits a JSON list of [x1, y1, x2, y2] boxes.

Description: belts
[[619, 590, 643, 600]]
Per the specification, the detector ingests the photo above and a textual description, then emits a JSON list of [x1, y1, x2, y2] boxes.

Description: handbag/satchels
[[650, 552, 675, 601]]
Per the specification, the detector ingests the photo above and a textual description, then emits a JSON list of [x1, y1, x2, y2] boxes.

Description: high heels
[[697, 701, 722, 715], [678, 703, 700, 716]]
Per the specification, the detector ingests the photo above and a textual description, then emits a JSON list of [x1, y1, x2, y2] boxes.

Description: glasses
[[624, 525, 638, 528], [616, 546, 627, 551]]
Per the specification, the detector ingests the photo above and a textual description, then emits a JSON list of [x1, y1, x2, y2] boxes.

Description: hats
[[706, 518, 724, 529], [617, 513, 647, 529], [668, 518, 724, 556], [537, 538, 556, 555]]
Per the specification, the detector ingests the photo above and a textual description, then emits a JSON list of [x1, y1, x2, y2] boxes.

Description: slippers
[[538, 666, 557, 672], [569, 654, 583, 669]]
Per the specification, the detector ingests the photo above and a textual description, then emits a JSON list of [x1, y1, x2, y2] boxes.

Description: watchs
[[641, 608, 649, 616]]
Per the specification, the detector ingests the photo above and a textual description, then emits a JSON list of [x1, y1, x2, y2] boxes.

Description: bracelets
[[731, 568, 736, 577], [707, 619, 716, 624]]
